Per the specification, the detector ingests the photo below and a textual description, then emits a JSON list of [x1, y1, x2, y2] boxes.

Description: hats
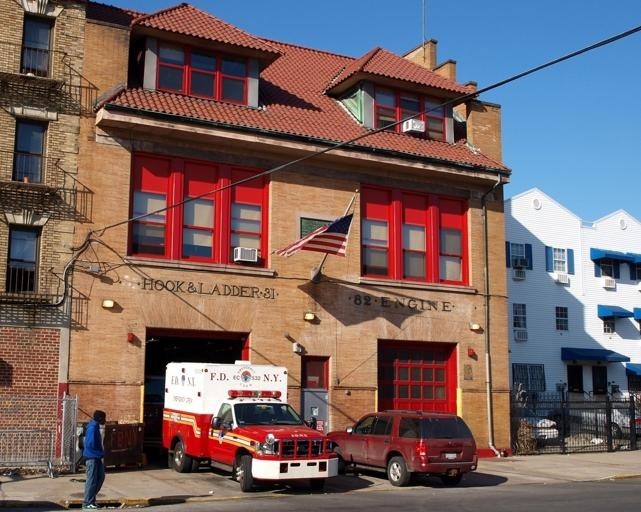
[[93, 410, 107, 422]]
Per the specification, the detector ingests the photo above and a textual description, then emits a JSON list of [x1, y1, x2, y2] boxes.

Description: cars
[[580, 402, 641, 440], [512, 408, 559, 450]]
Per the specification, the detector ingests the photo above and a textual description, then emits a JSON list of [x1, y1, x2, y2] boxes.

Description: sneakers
[[81, 503, 101, 510]]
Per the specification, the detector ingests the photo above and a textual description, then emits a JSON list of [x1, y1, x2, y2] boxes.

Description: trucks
[[160, 361, 341, 493]]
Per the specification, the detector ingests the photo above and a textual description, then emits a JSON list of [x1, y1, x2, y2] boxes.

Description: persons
[[82, 410, 107, 509]]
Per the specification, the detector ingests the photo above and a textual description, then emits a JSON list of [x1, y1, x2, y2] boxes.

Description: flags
[[270, 212, 355, 259]]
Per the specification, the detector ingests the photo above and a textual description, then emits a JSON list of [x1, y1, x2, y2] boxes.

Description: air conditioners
[[513, 269, 527, 280], [556, 273, 569, 284], [402, 118, 427, 135], [515, 330, 529, 341], [234, 246, 258, 263], [602, 278, 616, 289]]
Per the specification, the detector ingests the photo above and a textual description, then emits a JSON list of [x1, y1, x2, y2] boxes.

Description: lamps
[[469, 321, 482, 330], [102, 299, 115, 309], [303, 312, 316, 321]]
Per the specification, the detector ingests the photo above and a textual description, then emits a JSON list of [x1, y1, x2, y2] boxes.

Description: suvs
[[325, 409, 479, 489]]
